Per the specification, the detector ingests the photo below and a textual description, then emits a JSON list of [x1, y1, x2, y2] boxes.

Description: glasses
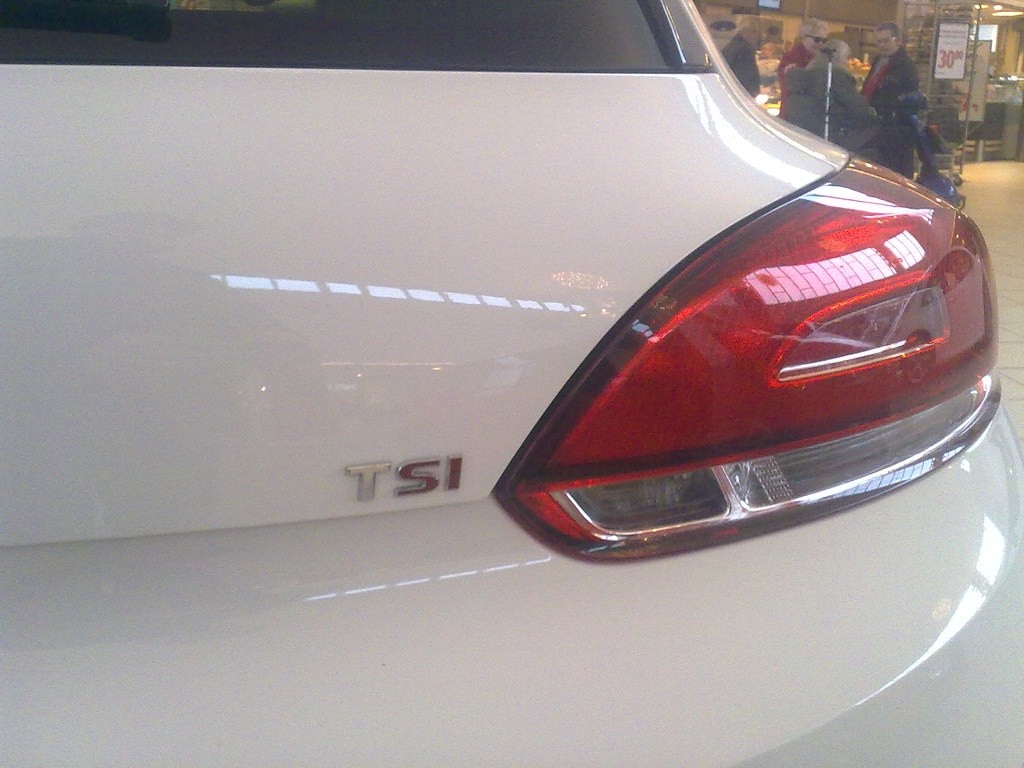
[[806, 35, 827, 43]]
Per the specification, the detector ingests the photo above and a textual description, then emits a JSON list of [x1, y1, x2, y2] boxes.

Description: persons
[[861, 23, 927, 179], [786, 39, 883, 165], [722, 18, 762, 99], [779, 18, 830, 117]]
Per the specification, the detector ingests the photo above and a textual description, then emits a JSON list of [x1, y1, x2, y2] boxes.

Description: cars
[[0, 0, 1024, 765]]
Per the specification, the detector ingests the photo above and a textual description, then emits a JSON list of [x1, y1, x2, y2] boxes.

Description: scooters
[[889, 90, 967, 210]]
